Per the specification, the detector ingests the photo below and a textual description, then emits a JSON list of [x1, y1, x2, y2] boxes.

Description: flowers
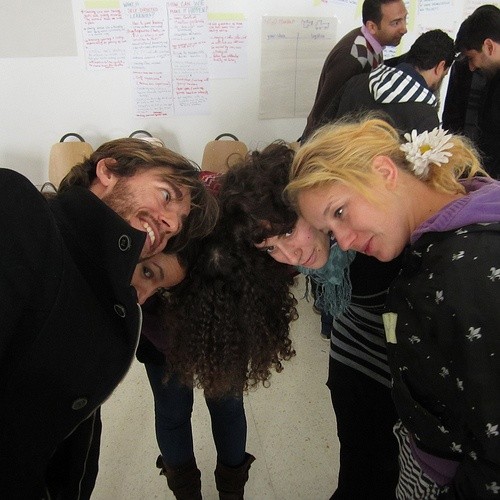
[[398, 126, 455, 175]]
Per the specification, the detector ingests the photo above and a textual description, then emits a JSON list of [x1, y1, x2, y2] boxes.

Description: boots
[[214, 451, 256, 499], [156, 452, 203, 499]]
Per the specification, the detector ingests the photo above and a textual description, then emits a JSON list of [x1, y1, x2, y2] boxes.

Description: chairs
[[48, 132, 94, 187], [200, 133, 248, 173]]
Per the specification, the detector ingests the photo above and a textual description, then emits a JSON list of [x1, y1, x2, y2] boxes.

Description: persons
[[280, 107, 500, 500], [131, 235, 210, 304], [219, 137, 401, 500], [298, 0, 408, 315], [320, 29, 455, 339], [441, 5, 500, 182], [0, 138, 208, 500], [455, 10, 500, 73], [135, 143, 299, 500]]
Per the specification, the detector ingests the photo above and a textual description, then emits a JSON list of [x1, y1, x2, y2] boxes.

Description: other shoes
[[320, 333, 330, 341]]
